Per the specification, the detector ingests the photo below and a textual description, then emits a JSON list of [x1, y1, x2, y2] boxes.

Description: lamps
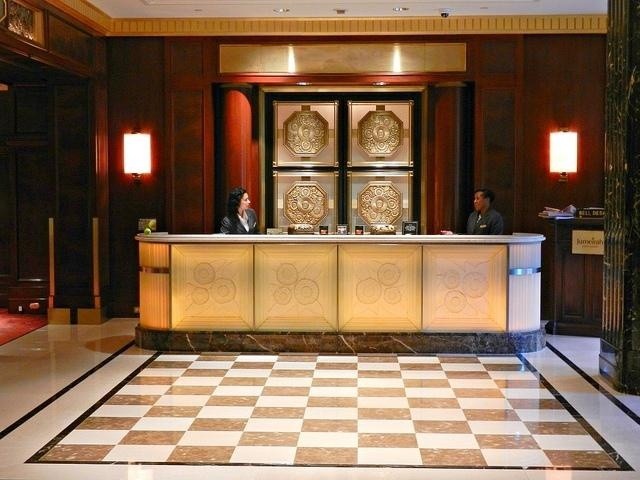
[[123, 127, 152, 185], [549, 127, 578, 184]]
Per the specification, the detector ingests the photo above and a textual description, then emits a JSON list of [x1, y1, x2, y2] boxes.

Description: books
[[536, 211, 573, 217], [542, 216, 574, 220]]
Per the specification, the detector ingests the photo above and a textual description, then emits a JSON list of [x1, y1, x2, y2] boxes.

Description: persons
[[466, 188, 504, 235], [218, 187, 258, 234]]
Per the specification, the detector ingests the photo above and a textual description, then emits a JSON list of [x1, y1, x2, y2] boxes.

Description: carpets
[[1, 303, 49, 351]]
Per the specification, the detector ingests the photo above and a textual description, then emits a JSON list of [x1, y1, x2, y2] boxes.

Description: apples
[[144, 228, 151, 235]]
